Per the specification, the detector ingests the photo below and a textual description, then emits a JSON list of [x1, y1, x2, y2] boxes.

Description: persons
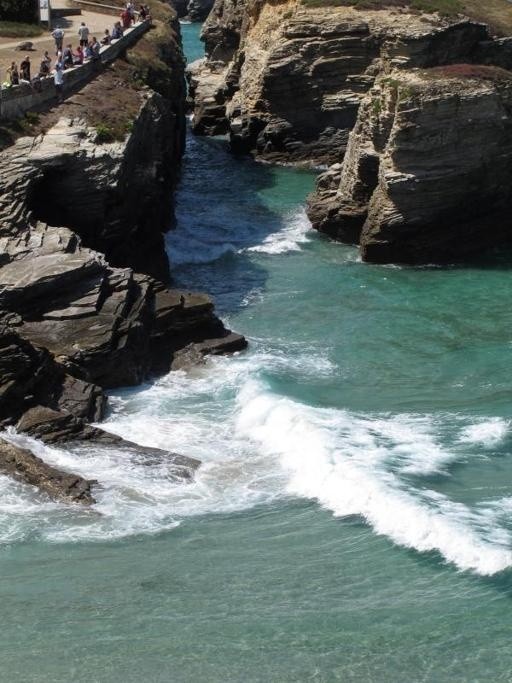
[[53, 64, 65, 105], [5, 0, 147, 86]]
